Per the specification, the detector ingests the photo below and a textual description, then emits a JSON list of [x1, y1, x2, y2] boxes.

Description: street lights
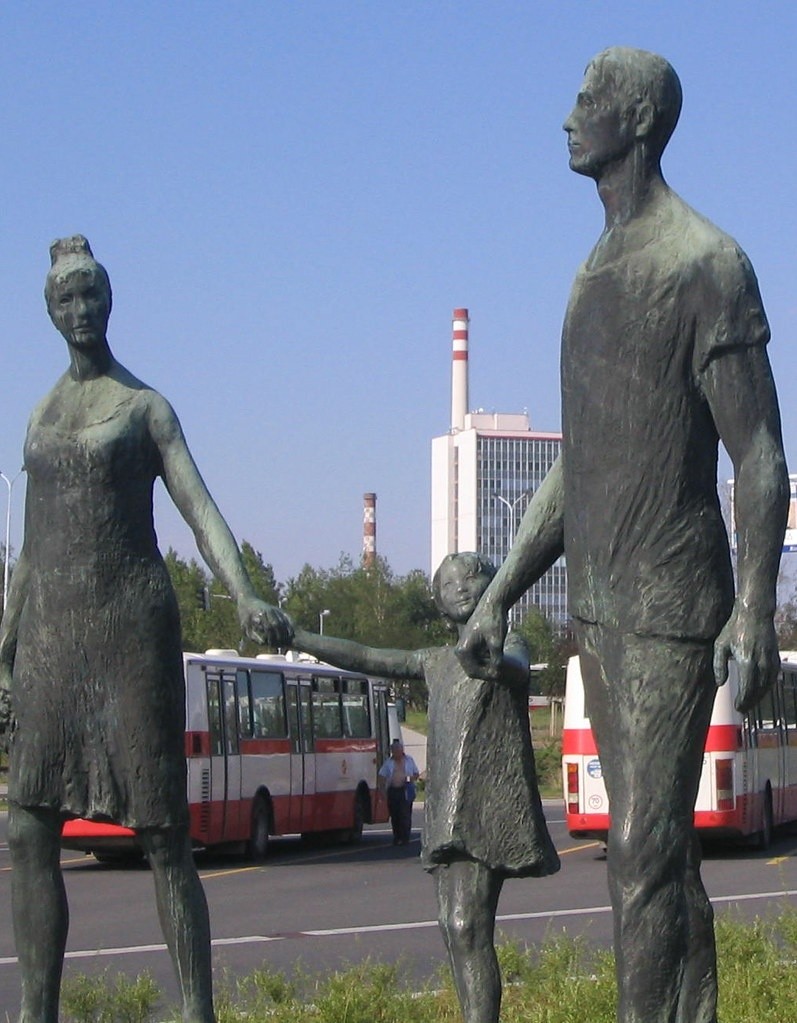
[[491, 488, 533, 634], [0, 465, 27, 611], [278, 598, 287, 654], [320, 609, 330, 635]]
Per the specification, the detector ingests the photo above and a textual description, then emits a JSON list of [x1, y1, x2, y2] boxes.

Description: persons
[[254, 551, 561, 1023], [0, 234, 293, 1023], [377, 742, 420, 845], [456, 48, 791, 1022]]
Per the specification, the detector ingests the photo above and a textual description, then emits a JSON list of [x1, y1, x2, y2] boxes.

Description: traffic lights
[[196, 587, 211, 612]]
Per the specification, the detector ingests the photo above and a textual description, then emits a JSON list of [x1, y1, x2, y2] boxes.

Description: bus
[[561, 650, 797, 854], [61, 649, 408, 867], [526, 663, 568, 713]]
